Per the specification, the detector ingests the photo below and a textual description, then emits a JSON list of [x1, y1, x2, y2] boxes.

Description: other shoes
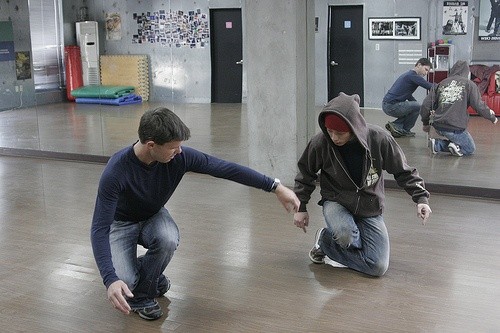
[[403, 128, 416, 137], [385, 121, 403, 138], [138, 255, 171, 295], [132, 302, 163, 321], [428, 137, 439, 154], [448, 143, 463, 157]]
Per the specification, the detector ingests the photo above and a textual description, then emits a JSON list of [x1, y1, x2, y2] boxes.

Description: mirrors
[[305, 0, 500, 190], [0, 0, 249, 167]]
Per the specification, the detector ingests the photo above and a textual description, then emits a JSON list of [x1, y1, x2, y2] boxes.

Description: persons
[[292, 91, 432, 277], [90, 108, 301, 320], [420, 60, 498, 156], [380, 57, 439, 137]]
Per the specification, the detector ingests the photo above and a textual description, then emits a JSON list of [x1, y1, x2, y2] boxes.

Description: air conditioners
[[75, 20, 100, 85]]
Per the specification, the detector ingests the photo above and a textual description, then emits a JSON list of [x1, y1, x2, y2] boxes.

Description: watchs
[[271, 176, 280, 191]]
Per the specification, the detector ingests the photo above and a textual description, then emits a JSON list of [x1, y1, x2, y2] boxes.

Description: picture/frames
[[368, 17, 422, 41]]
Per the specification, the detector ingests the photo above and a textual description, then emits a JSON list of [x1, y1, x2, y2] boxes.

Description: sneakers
[[308, 228, 327, 263]]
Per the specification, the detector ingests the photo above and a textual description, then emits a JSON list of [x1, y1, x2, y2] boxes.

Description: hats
[[325, 115, 350, 132]]
